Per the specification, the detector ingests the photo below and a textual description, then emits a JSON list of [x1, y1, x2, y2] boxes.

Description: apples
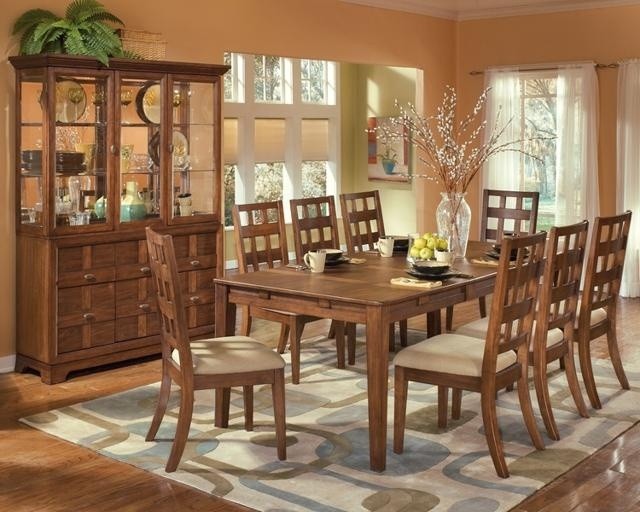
[[409, 232, 448, 260]]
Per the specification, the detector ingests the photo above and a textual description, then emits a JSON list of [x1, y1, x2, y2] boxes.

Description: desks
[[213, 240, 559, 472]]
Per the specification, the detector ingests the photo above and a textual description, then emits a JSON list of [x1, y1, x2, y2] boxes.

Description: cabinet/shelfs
[[8, 56, 231, 385]]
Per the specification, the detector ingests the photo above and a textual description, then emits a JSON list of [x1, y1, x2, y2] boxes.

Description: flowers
[[361, 84, 558, 255]]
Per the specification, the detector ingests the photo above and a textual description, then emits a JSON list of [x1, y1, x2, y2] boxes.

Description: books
[[389, 277, 443, 289]]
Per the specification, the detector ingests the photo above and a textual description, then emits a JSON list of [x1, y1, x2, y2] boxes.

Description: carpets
[[17, 323, 639, 512]]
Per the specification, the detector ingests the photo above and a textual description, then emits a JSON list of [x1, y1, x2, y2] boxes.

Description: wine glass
[[89, 90, 105, 124], [172, 90, 184, 126], [118, 89, 133, 125], [67, 87, 87, 124]]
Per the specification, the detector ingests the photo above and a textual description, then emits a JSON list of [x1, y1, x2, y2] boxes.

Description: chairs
[[328, 190, 409, 345], [393, 230, 548, 479], [276, 195, 357, 366], [534, 209, 632, 409], [445, 188, 540, 333], [450, 218, 590, 442], [145, 226, 286, 473], [230, 200, 345, 385]]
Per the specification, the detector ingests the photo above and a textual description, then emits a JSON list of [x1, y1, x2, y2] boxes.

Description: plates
[[324, 258, 348, 266], [406, 268, 457, 281], [142, 85, 168, 125], [487, 250, 528, 262], [21, 149, 88, 175], [49, 81, 88, 123], [388, 244, 409, 252], [149, 127, 190, 166]]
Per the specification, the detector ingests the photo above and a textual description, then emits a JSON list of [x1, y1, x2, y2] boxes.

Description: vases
[[381, 160, 395, 175], [435, 189, 472, 260]]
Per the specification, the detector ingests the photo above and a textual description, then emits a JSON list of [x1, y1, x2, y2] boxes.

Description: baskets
[[116, 28, 162, 41], [118, 38, 169, 60]]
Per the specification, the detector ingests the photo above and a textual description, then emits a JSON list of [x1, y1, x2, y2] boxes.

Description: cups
[[73, 142, 98, 172], [177, 193, 193, 216], [120, 143, 135, 173], [377, 238, 394, 258], [437, 248, 457, 266], [303, 250, 326, 272]]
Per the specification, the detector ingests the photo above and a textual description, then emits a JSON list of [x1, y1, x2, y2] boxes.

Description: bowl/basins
[[324, 248, 344, 259], [383, 235, 410, 245], [412, 260, 450, 274], [493, 242, 527, 255]]
